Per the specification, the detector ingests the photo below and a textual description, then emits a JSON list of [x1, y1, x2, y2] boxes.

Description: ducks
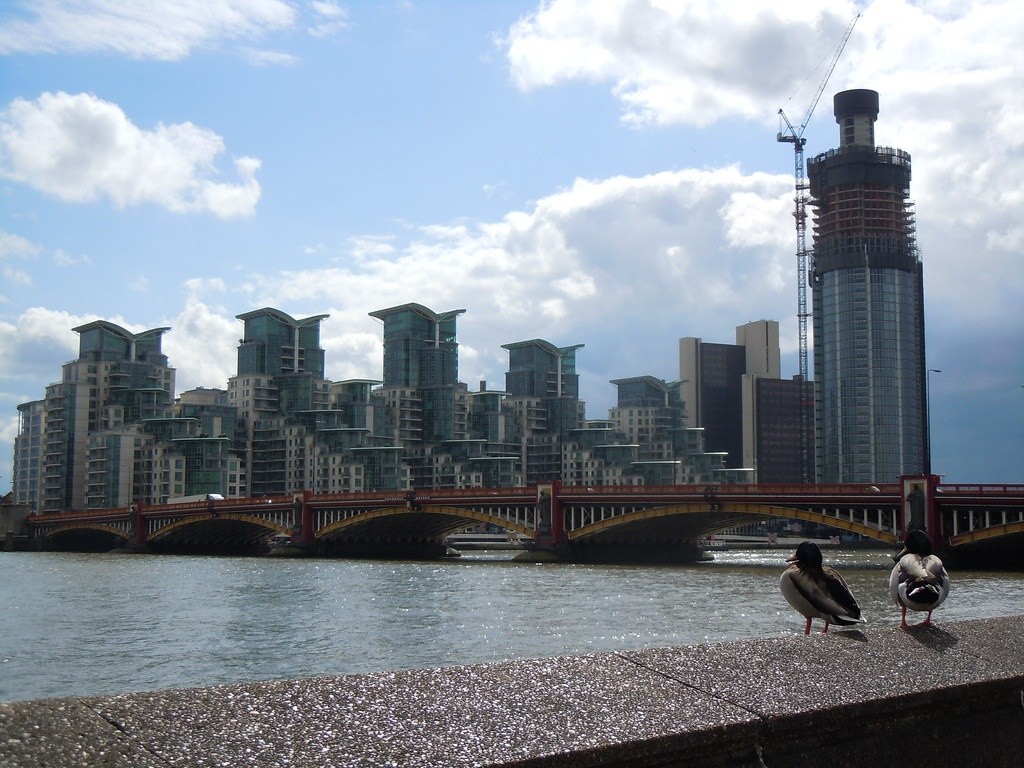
[[889, 529, 950, 628], [778, 541, 868, 636]]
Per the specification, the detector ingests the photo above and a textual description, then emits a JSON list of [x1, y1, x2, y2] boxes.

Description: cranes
[[774, 8, 863, 485]]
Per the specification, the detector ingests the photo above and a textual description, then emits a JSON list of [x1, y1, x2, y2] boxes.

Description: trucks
[[166, 493, 224, 504]]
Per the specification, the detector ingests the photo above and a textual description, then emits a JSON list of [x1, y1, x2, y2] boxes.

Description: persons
[[129, 506, 137, 531], [906, 484, 925, 531], [291, 497, 302, 528], [538, 491, 551, 529]]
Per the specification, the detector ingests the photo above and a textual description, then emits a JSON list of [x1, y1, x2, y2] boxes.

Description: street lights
[[926, 368, 942, 477]]
